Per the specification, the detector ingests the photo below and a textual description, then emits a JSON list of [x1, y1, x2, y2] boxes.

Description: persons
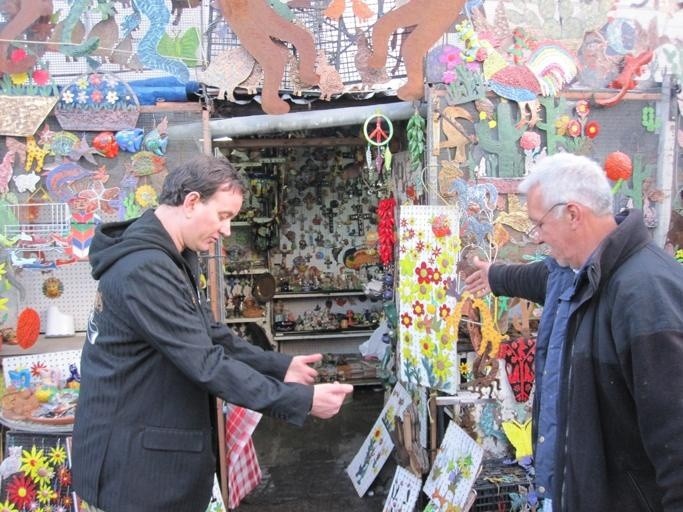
[[464, 153, 683, 512], [69, 153, 354, 512]]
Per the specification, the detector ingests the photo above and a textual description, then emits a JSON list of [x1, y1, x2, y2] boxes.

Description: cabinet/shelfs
[[214, 145, 286, 350], [271, 286, 385, 388]]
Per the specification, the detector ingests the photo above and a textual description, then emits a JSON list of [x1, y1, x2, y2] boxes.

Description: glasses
[[525, 202, 567, 241]]
